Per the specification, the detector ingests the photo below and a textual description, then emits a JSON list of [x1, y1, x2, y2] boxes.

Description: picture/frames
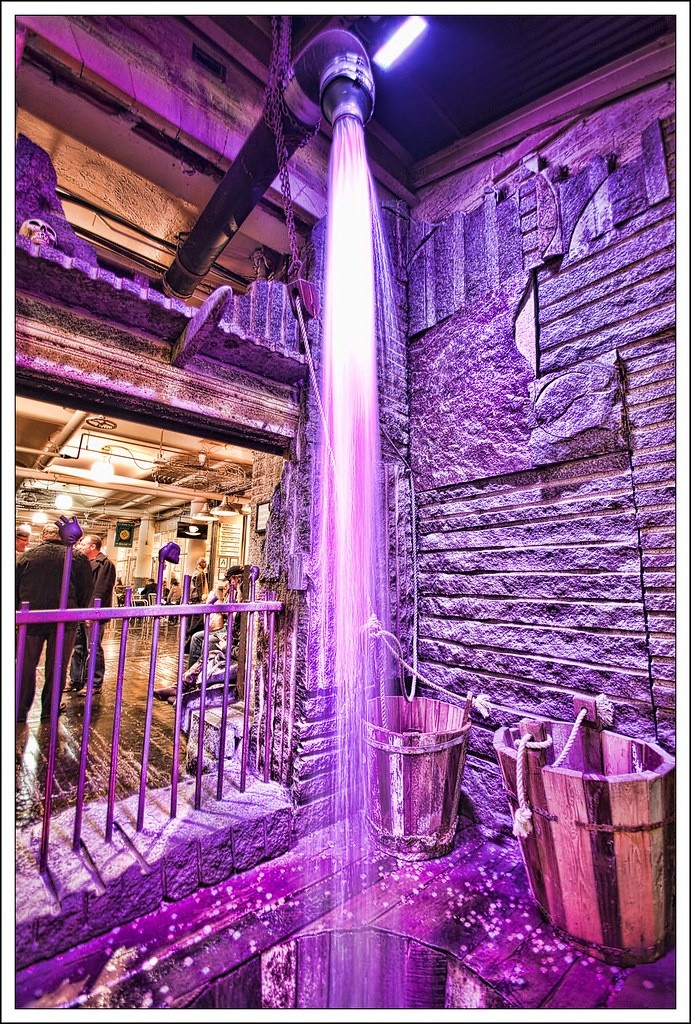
[[218, 556, 228, 568], [254, 500, 273, 534], [230, 557, 240, 568], [218, 567, 228, 580]]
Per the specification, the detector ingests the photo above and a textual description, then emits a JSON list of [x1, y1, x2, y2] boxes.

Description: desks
[[133, 594, 145, 599]]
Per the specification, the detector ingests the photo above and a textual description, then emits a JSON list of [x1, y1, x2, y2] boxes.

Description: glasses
[[16, 535, 28, 542]]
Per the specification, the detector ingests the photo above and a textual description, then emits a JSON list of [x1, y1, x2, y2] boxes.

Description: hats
[[222, 565, 244, 581]]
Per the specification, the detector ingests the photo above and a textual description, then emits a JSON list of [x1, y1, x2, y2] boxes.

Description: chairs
[[114, 585, 182, 627]]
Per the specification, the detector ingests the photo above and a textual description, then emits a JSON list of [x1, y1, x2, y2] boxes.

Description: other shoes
[[153, 685, 177, 705]]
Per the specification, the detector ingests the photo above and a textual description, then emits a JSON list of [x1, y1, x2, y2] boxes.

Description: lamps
[[197, 449, 207, 466], [95, 499, 112, 522], [24, 492, 38, 502], [191, 464, 219, 522], [240, 469, 252, 516], [174, 501, 191, 518], [210, 461, 239, 516]]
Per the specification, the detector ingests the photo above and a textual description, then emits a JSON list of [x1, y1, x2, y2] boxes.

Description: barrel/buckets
[[353, 690, 474, 862], [492, 695, 676, 965]]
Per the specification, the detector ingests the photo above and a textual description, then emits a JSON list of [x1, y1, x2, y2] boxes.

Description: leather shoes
[[62, 683, 79, 691], [78, 683, 102, 695]]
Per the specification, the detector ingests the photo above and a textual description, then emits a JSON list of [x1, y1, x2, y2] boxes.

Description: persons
[[116, 557, 248, 701], [15, 524, 31, 560], [64, 534, 116, 698], [15, 521, 95, 723]]
